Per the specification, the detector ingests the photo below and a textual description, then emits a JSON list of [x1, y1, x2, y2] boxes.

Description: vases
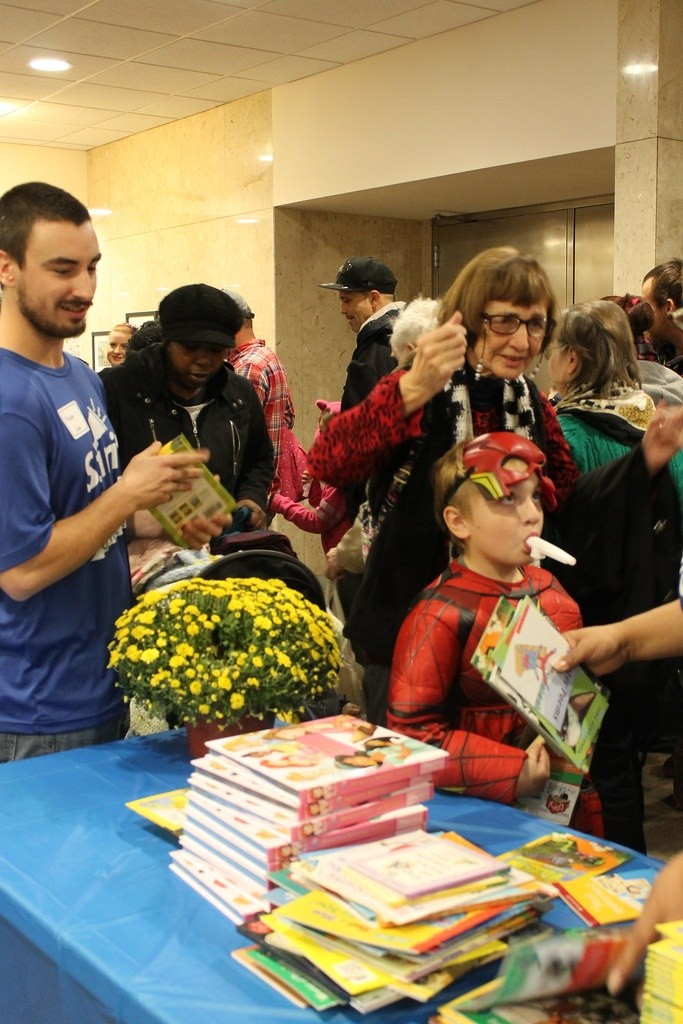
[[180, 712, 274, 759]]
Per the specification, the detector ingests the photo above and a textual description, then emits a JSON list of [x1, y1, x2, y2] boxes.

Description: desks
[[0, 728, 663, 1024]]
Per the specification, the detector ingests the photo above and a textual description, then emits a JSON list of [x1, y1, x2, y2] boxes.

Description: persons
[[219, 285, 295, 516], [305, 250, 582, 728], [0, 179, 234, 765], [108, 321, 136, 366], [388, 432, 610, 841], [303, 247, 683, 995], [98, 285, 276, 540], [267, 399, 351, 557]]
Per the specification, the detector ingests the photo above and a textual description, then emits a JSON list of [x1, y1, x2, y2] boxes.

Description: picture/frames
[[92, 331, 112, 372], [125, 310, 158, 328]]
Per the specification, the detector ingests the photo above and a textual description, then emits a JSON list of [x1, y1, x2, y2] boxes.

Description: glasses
[[543, 344, 565, 359], [480, 311, 557, 338]]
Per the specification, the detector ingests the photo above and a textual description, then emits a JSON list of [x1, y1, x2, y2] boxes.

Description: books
[[147, 434, 236, 549], [470, 595, 610, 772], [124, 715, 683, 1024]]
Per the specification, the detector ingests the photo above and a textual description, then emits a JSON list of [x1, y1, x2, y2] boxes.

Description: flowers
[[107, 577, 348, 730]]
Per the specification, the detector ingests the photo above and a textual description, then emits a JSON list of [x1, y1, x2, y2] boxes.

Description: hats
[[317, 256, 397, 294], [159, 283, 243, 348], [221, 289, 255, 317]]
